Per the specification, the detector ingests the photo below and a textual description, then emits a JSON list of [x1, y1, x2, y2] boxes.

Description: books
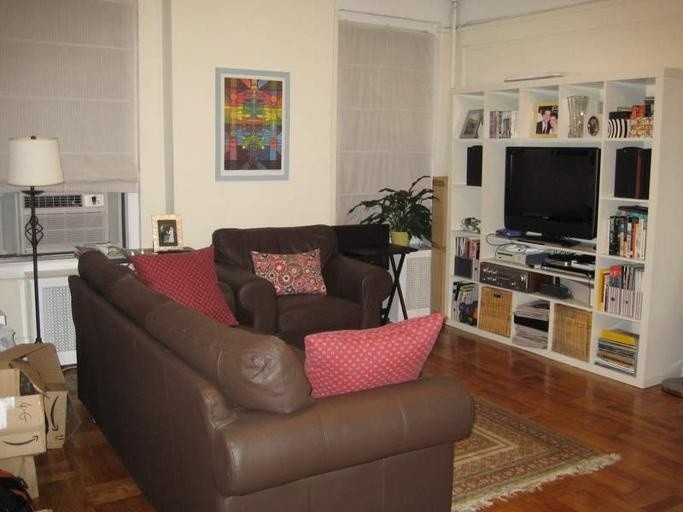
[[450, 235, 479, 321], [594, 205, 648, 377]]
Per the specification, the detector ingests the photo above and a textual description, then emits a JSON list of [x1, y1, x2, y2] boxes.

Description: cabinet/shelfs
[[380, 242, 418, 323], [430, 174, 450, 316], [440, 58, 682, 392]]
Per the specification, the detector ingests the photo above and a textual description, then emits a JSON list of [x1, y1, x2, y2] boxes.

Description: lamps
[[2, 131, 68, 343]]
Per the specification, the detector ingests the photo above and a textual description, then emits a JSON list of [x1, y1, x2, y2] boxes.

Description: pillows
[[248, 244, 332, 299], [298, 311, 451, 401], [125, 241, 239, 332]]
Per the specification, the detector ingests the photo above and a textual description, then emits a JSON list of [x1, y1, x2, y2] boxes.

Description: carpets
[[52, 362, 621, 510]]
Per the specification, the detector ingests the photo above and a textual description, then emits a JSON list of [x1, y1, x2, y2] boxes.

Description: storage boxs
[[0, 338, 73, 503]]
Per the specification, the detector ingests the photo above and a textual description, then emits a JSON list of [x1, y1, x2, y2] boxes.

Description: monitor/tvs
[[504, 146, 601, 247]]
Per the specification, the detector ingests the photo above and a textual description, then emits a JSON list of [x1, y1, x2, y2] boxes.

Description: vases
[[565, 93, 587, 139]]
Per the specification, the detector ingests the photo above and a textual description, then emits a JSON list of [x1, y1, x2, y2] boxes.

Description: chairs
[[203, 222, 396, 349]]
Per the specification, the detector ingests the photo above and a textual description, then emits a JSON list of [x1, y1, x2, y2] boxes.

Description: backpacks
[[0, 469, 36, 512]]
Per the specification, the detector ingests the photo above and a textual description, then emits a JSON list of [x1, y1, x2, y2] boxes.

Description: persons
[[160, 224, 174, 243], [549, 113, 558, 134], [536, 107, 552, 134]]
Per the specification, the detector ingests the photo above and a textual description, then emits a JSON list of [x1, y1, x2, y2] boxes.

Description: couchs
[[66, 231, 477, 511]]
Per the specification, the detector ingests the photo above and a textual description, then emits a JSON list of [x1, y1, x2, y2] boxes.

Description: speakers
[[614, 146, 652, 200], [467, 144, 482, 186]]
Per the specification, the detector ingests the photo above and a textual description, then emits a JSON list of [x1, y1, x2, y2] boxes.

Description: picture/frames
[[150, 212, 186, 255], [459, 108, 486, 140], [214, 66, 292, 184], [531, 100, 561, 140]]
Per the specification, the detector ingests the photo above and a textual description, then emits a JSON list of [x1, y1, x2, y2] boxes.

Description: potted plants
[[346, 172, 441, 248]]
